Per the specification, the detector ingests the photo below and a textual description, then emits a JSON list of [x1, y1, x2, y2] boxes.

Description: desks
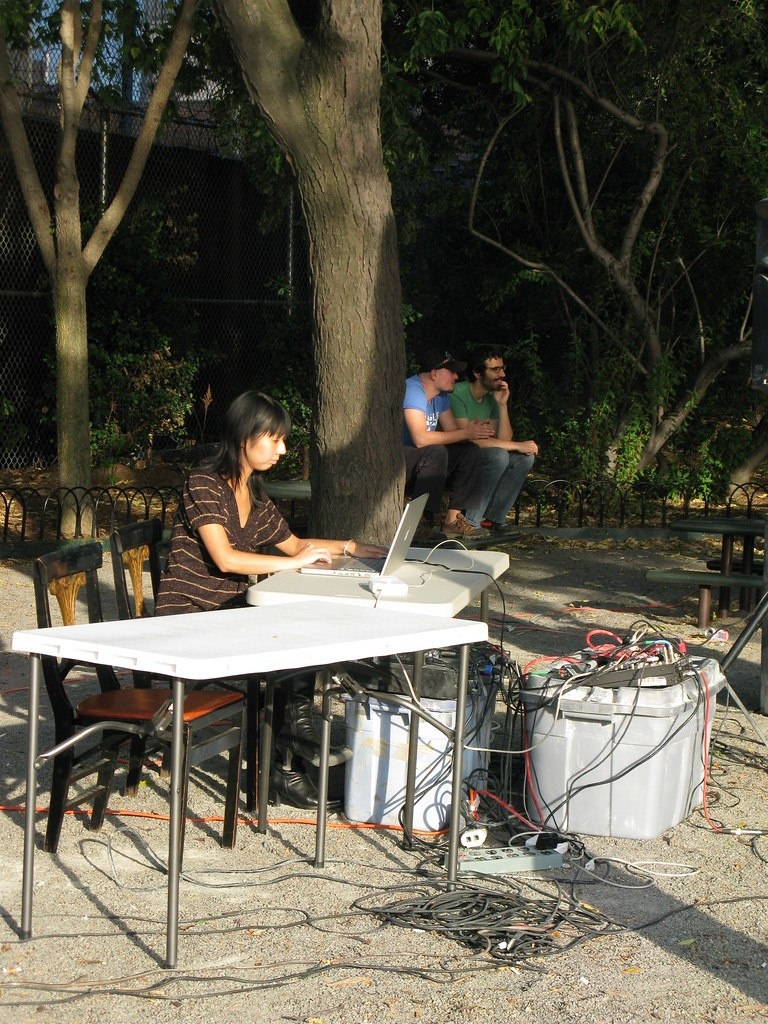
[[10, 601, 489, 970], [668, 518, 767, 618], [247, 548, 511, 835]]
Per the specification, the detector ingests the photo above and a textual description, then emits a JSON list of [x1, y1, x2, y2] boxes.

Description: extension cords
[[443, 845, 563, 875]]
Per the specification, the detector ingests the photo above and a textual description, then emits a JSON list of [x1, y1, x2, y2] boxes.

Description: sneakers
[[441, 513, 489, 539], [411, 517, 456, 546]]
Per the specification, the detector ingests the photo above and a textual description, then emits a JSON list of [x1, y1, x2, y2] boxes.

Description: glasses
[[486, 365, 506, 373]]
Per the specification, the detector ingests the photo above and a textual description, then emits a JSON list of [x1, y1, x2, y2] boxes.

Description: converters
[[369, 577, 409, 597]]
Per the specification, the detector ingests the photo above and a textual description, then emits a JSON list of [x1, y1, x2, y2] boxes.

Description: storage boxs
[[518, 644, 728, 840], [344, 675, 503, 831]]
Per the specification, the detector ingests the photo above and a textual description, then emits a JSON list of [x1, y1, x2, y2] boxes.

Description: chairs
[[34, 542, 247, 873], [109, 517, 307, 812]]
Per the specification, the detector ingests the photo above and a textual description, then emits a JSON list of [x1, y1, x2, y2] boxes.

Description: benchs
[[646, 569, 763, 630], [411, 531, 522, 625]]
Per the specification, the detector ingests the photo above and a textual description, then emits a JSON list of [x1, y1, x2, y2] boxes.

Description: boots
[[277, 670, 353, 770], [271, 691, 341, 810]]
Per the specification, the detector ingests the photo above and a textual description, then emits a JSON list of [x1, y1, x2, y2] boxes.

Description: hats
[[420, 344, 467, 371]]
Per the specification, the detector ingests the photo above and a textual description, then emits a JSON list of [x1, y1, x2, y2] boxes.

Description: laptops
[[300, 492, 430, 578]]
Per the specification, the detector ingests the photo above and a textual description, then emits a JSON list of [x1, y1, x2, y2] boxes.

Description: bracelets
[[344, 539, 354, 556]]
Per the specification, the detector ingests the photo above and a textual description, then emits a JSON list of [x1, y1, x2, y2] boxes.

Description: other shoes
[[476, 527, 493, 536], [481, 518, 509, 531]]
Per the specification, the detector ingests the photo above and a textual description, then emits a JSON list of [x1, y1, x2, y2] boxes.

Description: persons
[[156, 390, 388, 809], [402, 349, 491, 543], [449, 349, 538, 535]]
